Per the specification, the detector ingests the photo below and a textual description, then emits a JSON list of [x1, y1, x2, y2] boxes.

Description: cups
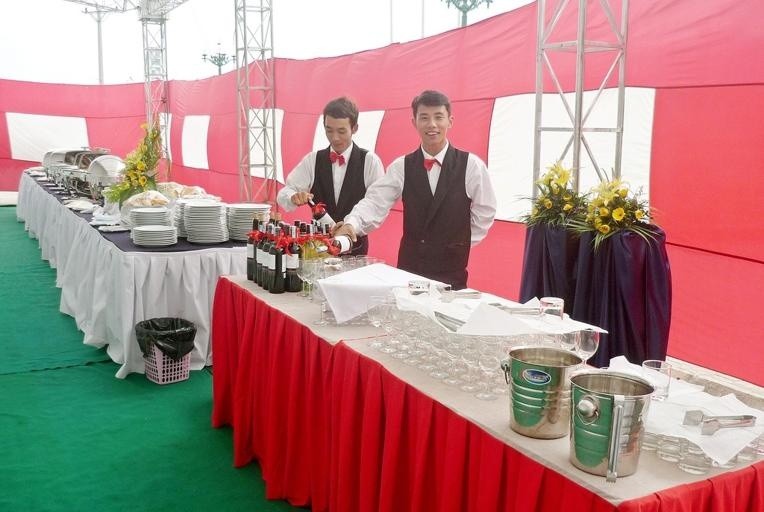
[[641, 359, 670, 403], [642, 427, 764, 476]]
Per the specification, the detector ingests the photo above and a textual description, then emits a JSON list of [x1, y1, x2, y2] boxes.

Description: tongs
[[682, 409, 756, 436], [605, 393, 626, 484]]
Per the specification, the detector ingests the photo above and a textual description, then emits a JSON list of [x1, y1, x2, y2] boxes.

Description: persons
[[274, 95, 387, 258], [332, 89, 498, 291]]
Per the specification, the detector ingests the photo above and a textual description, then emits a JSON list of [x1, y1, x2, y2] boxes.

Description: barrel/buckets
[[567, 371, 656, 481], [502, 345, 587, 441]]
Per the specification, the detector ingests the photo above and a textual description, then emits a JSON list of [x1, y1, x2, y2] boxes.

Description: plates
[[24, 164, 132, 235], [128, 197, 272, 247]]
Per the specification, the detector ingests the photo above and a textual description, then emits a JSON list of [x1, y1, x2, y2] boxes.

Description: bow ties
[[423, 159, 437, 170], [329, 151, 345, 166]]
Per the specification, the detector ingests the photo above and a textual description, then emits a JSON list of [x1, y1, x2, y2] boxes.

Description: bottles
[[294, 198, 336, 258], [316, 233, 363, 256], [245, 219, 299, 294]]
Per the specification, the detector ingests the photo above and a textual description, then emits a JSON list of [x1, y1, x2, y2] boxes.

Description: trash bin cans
[[135, 317, 197, 385]]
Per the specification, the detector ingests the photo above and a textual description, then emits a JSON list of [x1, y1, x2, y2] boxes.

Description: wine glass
[[294, 256, 601, 402]]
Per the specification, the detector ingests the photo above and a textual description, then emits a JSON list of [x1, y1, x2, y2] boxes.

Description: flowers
[[99, 122, 166, 210], [514, 158, 664, 256]]
